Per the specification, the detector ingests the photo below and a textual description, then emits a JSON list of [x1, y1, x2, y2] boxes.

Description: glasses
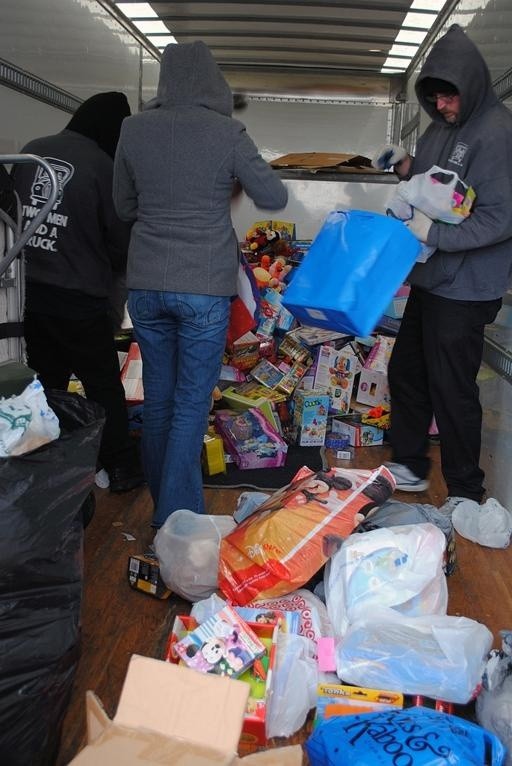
[[424, 95, 455, 103]]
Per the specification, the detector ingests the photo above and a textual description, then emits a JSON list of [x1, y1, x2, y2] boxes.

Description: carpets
[[201, 431, 328, 492]]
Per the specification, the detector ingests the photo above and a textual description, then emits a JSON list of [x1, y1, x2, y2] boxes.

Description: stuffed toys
[[246, 223, 280, 249], [328, 355, 351, 389], [247, 255, 283, 290], [269, 257, 292, 290]]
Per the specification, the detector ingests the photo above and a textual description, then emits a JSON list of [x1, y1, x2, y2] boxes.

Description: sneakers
[[108, 457, 146, 495], [382, 460, 431, 493], [439, 495, 479, 521]]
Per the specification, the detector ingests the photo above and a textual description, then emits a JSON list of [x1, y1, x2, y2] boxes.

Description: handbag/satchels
[[301, 705, 506, 766], [217, 464, 398, 607], [313, 502, 458, 603], [280, 210, 423, 339]]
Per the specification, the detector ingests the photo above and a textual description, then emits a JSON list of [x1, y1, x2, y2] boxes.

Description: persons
[[371, 22, 512, 525], [111, 40, 289, 532], [8, 91, 142, 494]]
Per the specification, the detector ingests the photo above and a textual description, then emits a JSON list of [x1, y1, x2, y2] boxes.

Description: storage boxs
[[66, 655, 304, 766]]
